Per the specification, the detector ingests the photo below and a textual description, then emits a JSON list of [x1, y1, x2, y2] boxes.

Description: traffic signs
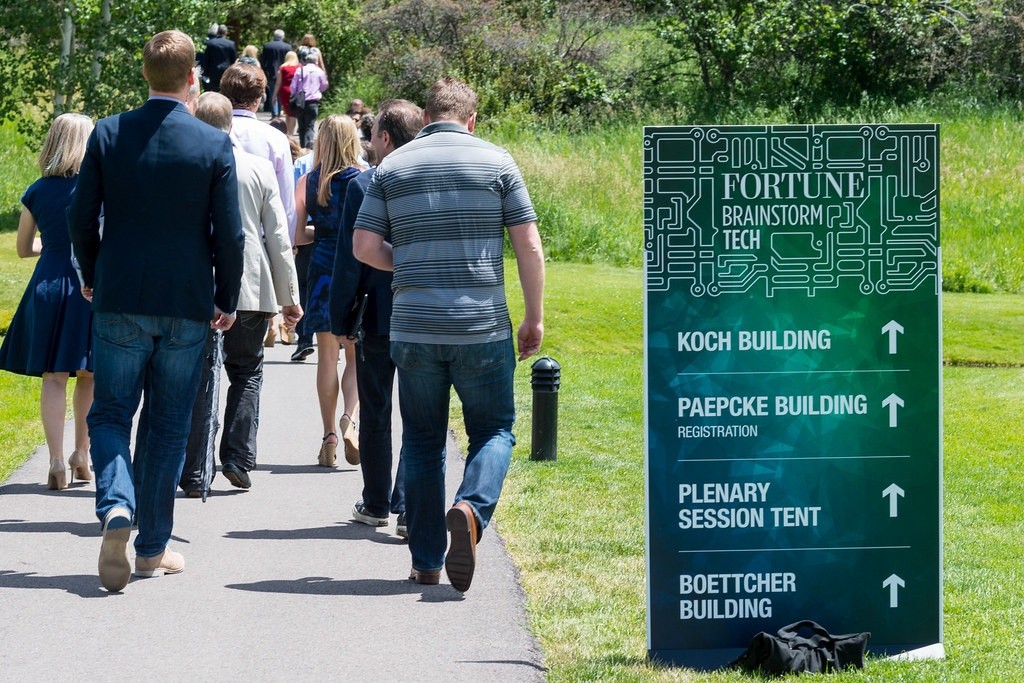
[[641, 121, 943, 664]]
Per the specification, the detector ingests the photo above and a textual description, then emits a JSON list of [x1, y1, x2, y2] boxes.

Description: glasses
[[352, 117, 359, 122]]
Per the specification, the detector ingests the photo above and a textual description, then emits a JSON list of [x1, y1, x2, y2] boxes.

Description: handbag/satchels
[[347, 291, 370, 340], [288, 92, 305, 113], [718, 620, 871, 675]]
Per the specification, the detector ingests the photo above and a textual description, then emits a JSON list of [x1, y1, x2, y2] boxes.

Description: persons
[[352, 78, 544, 592], [179, 23, 424, 496], [67, 31, 245, 591], [0, 113, 94, 491]]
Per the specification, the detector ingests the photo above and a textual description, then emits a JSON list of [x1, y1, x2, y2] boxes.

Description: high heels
[[68, 451, 92, 484], [340, 414, 360, 465], [318, 432, 338, 466], [46, 461, 67, 491]]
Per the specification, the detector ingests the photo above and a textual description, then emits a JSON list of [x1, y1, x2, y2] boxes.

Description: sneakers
[[353, 499, 388, 527], [134, 548, 185, 577], [395, 512, 408, 537], [221, 463, 251, 488], [290, 339, 314, 361], [98, 506, 132, 591], [188, 486, 211, 497]]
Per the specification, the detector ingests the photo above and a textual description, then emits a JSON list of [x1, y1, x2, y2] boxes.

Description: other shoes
[[279, 321, 291, 345], [265, 329, 278, 347]]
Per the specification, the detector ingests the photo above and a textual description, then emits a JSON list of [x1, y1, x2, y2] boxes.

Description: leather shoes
[[446, 502, 477, 593], [410, 568, 440, 585]]
